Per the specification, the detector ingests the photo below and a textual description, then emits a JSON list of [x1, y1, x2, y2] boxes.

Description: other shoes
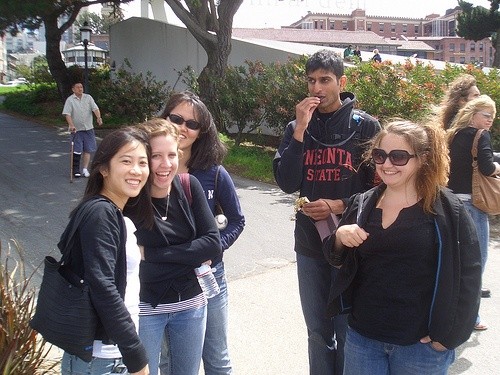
[[473, 325, 487, 329], [82, 168, 90, 178], [481, 291, 491, 296]]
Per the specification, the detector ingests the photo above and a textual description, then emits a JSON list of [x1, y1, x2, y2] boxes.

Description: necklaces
[[152, 185, 171, 221]]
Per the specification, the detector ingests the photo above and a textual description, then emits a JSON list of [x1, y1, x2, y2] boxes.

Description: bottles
[[194, 262, 220, 299], [214, 214, 228, 230]]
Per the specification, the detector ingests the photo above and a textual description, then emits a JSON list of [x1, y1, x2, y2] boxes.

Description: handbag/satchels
[[339, 191, 365, 314], [471, 128, 500, 214], [29, 199, 122, 362]]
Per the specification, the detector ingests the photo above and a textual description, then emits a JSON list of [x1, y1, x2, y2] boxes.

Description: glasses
[[168, 113, 202, 130], [372, 148, 419, 167]]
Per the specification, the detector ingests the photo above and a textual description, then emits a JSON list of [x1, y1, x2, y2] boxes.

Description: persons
[[29, 126, 153, 375], [324, 119, 483, 375], [156, 91, 245, 375], [343, 44, 382, 65], [273, 50, 380, 375], [62, 82, 103, 178], [122, 118, 223, 375], [437, 73, 500, 330]]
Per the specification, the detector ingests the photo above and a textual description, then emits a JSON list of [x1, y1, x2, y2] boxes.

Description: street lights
[[79, 21, 93, 94]]
